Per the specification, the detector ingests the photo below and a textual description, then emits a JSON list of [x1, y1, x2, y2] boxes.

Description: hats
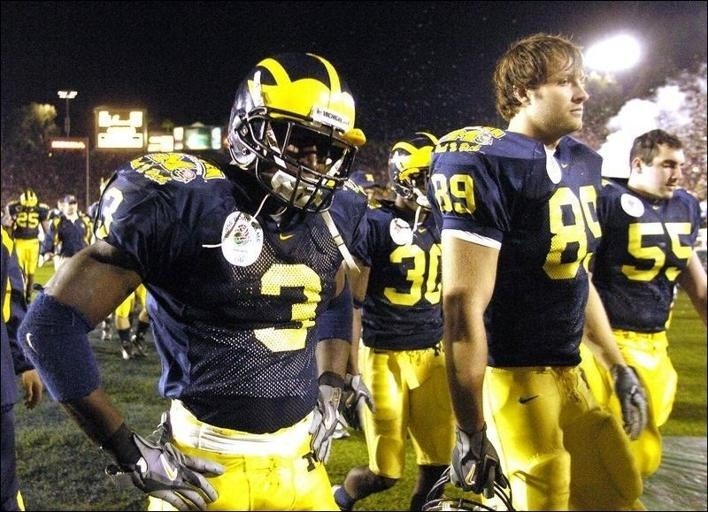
[[63, 195, 79, 206]]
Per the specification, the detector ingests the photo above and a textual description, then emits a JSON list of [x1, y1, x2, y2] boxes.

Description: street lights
[[56, 90, 79, 136]]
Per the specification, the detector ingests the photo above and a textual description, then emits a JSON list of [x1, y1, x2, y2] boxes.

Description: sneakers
[[120, 341, 135, 361], [131, 334, 151, 358], [99, 323, 115, 343], [331, 484, 356, 512]]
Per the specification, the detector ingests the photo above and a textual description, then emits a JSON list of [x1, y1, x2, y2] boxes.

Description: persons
[[330, 125, 456, 511], [0, 228, 44, 511], [595, 127, 708, 432], [16, 49, 372, 512], [429, 28, 646, 512]]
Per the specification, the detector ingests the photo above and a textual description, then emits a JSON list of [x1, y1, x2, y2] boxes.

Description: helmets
[[20, 188, 38, 207], [387, 130, 438, 213], [227, 51, 369, 214]]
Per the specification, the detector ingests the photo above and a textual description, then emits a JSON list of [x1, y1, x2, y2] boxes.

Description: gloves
[[105, 420, 223, 511], [608, 364, 648, 442], [345, 372, 376, 429], [307, 370, 348, 464], [448, 424, 507, 499]]
